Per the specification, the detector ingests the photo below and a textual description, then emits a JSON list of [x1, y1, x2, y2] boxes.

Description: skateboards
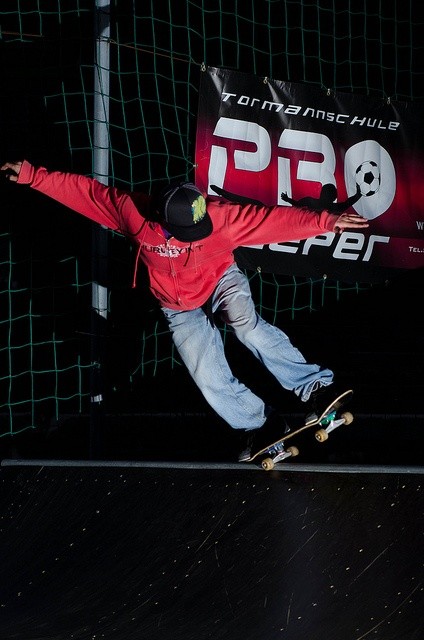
[[240, 388, 355, 471]]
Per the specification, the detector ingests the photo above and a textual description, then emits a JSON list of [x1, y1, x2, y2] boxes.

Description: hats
[[155, 181, 212, 242]]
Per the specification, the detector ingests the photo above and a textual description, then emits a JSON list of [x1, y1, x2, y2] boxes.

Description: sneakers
[[238, 407, 291, 462], [305, 382, 337, 425]]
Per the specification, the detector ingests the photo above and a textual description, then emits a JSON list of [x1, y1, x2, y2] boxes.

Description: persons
[[281, 184, 363, 280], [0, 161, 370, 460]]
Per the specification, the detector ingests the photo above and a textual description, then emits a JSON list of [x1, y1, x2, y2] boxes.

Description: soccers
[[355, 161, 380, 197]]
